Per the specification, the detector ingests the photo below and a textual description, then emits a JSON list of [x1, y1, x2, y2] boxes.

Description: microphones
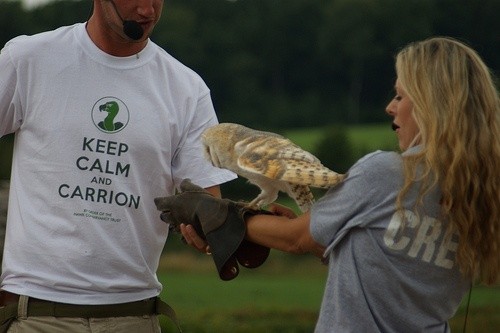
[[110, 0, 144, 40], [392, 123, 400, 130]]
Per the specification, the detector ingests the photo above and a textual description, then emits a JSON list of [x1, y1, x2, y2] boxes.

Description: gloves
[[154, 178, 276, 281]]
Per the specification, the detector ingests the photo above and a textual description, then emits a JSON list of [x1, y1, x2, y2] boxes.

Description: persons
[[0, 0, 239, 332], [153, 36, 500, 332]]
